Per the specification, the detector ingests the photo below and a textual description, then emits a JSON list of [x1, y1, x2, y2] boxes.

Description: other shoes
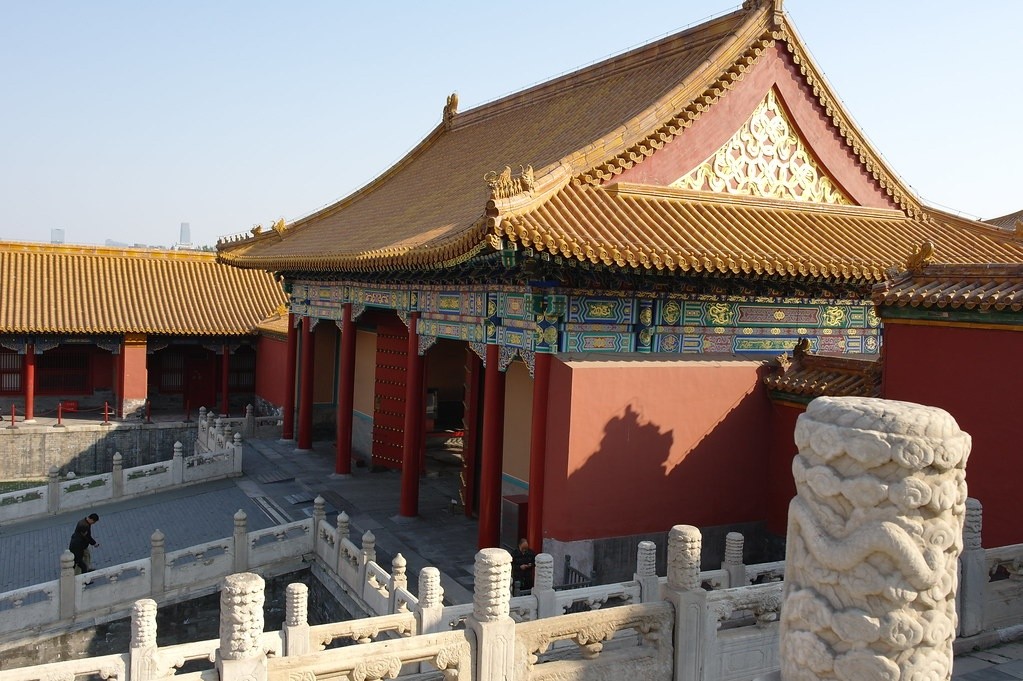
[[85, 582, 94, 585], [87, 568, 95, 572]]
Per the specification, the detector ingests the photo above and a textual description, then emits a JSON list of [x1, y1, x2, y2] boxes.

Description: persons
[[510, 538, 537, 598], [68, 513, 99, 584]]
